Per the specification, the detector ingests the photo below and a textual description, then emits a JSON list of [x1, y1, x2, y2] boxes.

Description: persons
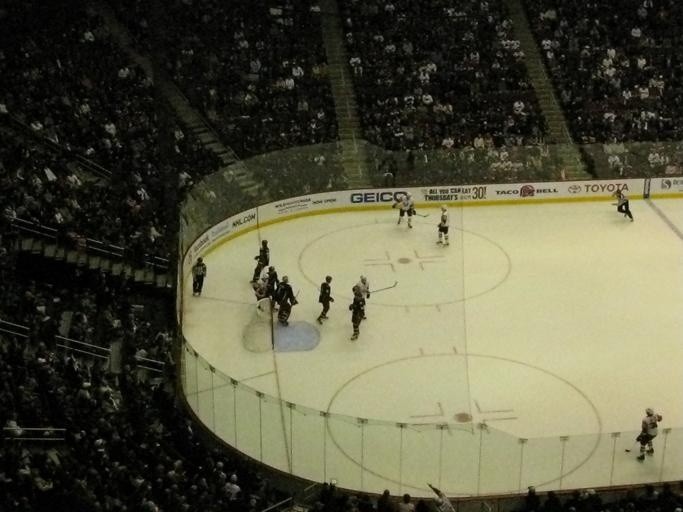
[[348, 275, 371, 341], [317, 275, 334, 325], [175, 1, 681, 245], [192, 257, 207, 296], [252, 266, 298, 326], [1, 2, 182, 511], [250, 239, 270, 284], [178, 394, 682, 512]]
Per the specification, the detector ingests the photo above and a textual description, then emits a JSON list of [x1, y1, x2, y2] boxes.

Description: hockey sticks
[[394, 206, 429, 217], [371, 281, 397, 292], [625, 440, 638, 451]]
